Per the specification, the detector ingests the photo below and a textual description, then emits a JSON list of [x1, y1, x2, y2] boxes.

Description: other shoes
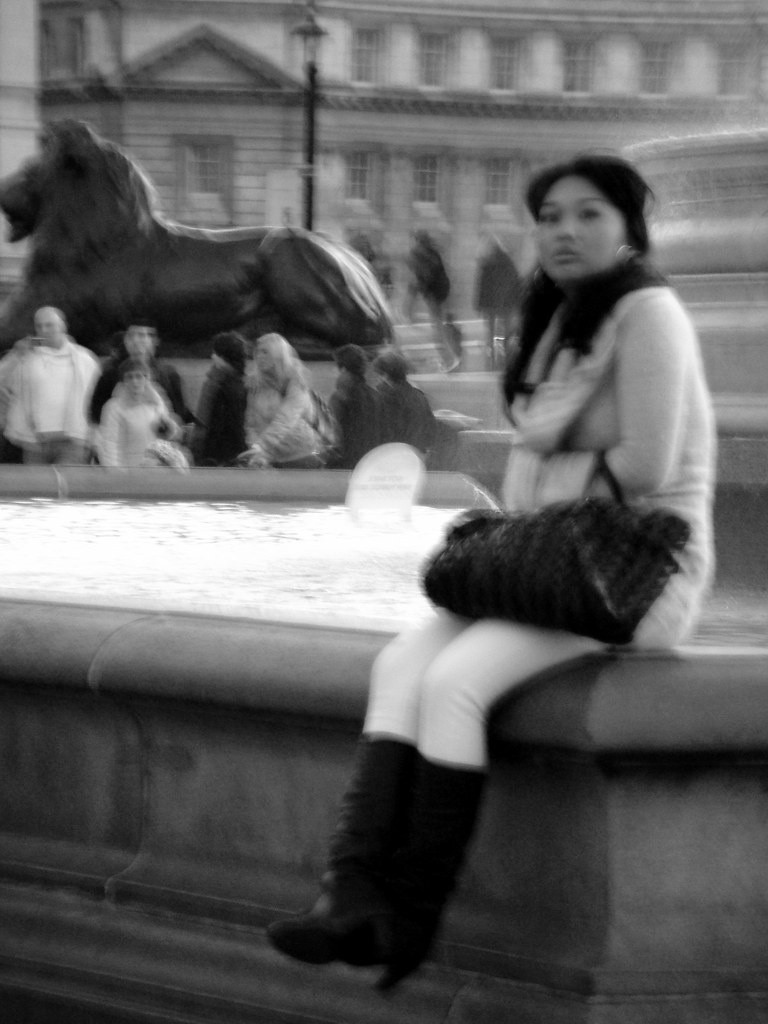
[[439, 356, 460, 374]]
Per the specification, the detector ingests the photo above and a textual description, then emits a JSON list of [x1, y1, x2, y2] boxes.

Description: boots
[[269, 734, 486, 999]]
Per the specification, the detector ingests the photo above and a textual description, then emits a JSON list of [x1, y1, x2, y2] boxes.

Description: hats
[[333, 344, 367, 373], [214, 331, 247, 375]]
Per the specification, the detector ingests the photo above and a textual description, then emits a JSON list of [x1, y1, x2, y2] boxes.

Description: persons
[[268, 151, 718, 998], [0, 220, 523, 470]]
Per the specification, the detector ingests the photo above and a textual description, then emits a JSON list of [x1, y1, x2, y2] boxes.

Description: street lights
[[289, 10, 329, 233]]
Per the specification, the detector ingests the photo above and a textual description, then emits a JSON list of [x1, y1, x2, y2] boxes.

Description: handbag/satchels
[[423, 452, 690, 645], [311, 392, 336, 446]]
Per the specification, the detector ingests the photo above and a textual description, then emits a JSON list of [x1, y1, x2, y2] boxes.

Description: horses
[[0, 119, 396, 355]]
